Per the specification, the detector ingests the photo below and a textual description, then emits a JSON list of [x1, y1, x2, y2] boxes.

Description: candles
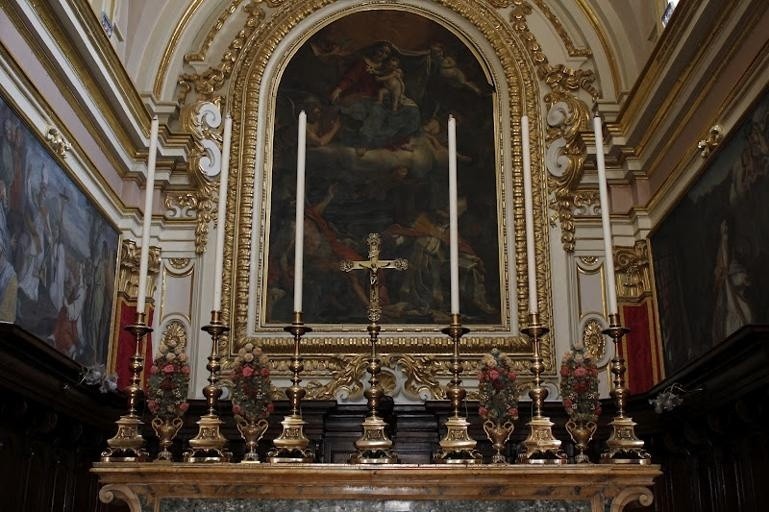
[[592, 110, 619, 314], [520, 111, 539, 315], [136, 108, 159, 316], [446, 110, 459, 314], [292, 106, 307, 312], [210, 109, 234, 313]]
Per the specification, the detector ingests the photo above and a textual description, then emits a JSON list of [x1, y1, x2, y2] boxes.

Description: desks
[[87, 457, 663, 511]]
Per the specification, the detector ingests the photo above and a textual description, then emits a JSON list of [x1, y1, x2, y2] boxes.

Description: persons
[[0, 114, 118, 374], [710, 218, 752, 346]]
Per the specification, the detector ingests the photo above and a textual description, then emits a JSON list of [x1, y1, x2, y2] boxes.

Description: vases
[[566, 419, 599, 464], [152, 417, 184, 460], [482, 419, 515, 462], [236, 419, 268, 462]]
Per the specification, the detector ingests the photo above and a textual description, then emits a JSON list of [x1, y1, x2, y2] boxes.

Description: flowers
[[147, 339, 191, 418], [230, 344, 274, 423], [476, 349, 522, 422], [561, 339, 601, 425]]
